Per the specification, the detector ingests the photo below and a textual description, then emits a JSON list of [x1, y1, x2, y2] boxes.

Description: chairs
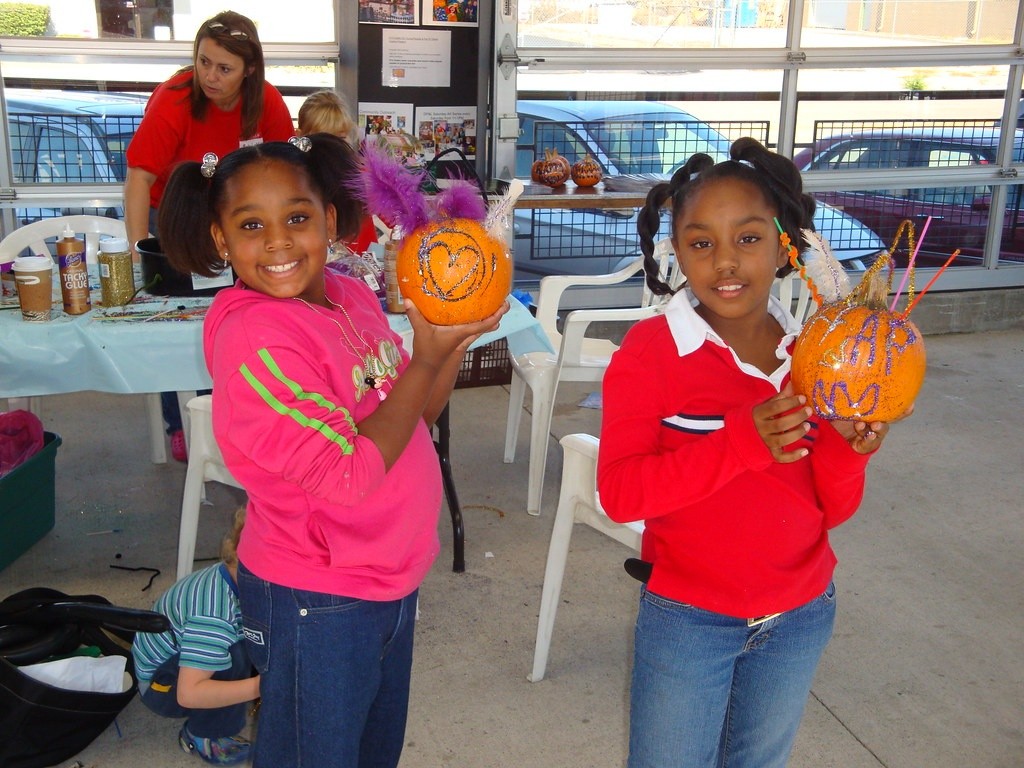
[[176, 330, 411, 582], [500, 225, 839, 677]]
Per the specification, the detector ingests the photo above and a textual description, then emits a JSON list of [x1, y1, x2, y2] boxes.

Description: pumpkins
[[530, 147, 603, 188], [396, 208, 512, 327], [790, 269, 925, 424]]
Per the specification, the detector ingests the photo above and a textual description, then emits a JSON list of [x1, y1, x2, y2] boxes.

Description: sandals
[[178, 719, 251, 765]]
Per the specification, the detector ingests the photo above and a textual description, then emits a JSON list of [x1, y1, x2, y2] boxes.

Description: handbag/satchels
[[414, 148, 488, 204], [0, 587, 166, 768]]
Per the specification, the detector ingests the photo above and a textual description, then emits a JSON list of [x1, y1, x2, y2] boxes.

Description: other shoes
[[171, 430, 187, 460]]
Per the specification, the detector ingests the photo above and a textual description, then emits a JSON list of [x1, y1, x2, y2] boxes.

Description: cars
[[0, 87, 153, 265], [789, 99, 1023, 270], [513, 99, 893, 290]]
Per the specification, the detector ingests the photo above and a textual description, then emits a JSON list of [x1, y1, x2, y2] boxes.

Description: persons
[[419, 122, 475, 155], [132, 505, 262, 765], [596, 138, 916, 768], [157, 132, 510, 768], [298, 92, 399, 255], [124, 12, 295, 464]]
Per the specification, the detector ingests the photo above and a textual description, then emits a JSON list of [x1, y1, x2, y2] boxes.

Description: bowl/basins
[[135, 237, 240, 297]]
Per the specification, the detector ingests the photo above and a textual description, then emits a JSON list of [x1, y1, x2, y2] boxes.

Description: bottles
[[97, 239, 135, 308], [56, 222, 91, 315], [385, 225, 408, 315]]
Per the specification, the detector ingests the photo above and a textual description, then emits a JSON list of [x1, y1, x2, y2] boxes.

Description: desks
[[2, 254, 554, 571]]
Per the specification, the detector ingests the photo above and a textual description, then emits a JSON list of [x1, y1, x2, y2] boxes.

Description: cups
[[12, 257, 53, 322]]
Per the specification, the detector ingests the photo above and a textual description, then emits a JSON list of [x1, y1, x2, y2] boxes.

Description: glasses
[[208, 21, 248, 42]]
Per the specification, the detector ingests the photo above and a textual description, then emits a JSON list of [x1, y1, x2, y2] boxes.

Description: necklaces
[[294, 294, 377, 389]]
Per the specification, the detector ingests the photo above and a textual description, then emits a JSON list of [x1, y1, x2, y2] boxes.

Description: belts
[[624, 558, 781, 627]]
